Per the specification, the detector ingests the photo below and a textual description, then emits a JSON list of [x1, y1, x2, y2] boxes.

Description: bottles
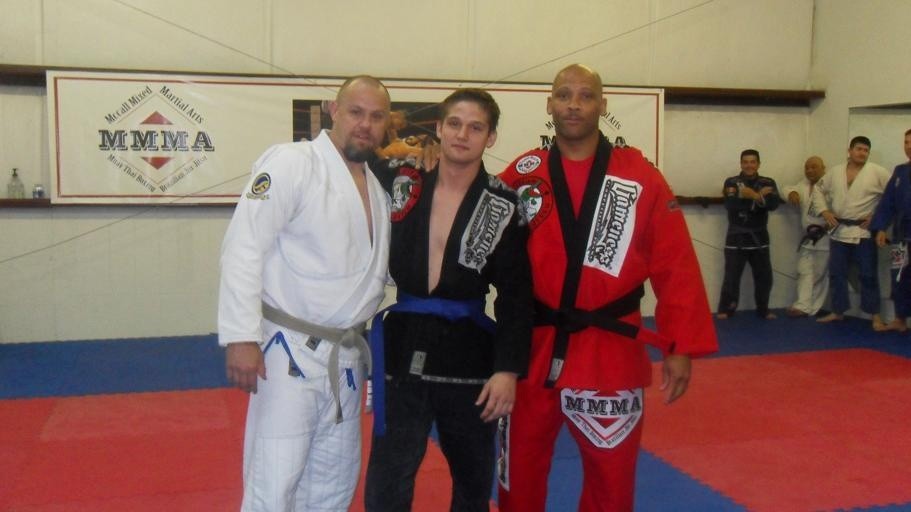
[[8, 169, 24, 199]]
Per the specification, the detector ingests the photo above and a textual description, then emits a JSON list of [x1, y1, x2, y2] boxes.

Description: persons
[[415, 61, 722, 512], [871, 128, 911, 335], [364, 87, 536, 512], [811, 136, 891, 330], [716, 148, 783, 322], [214, 74, 396, 512], [781, 155, 835, 318]]
